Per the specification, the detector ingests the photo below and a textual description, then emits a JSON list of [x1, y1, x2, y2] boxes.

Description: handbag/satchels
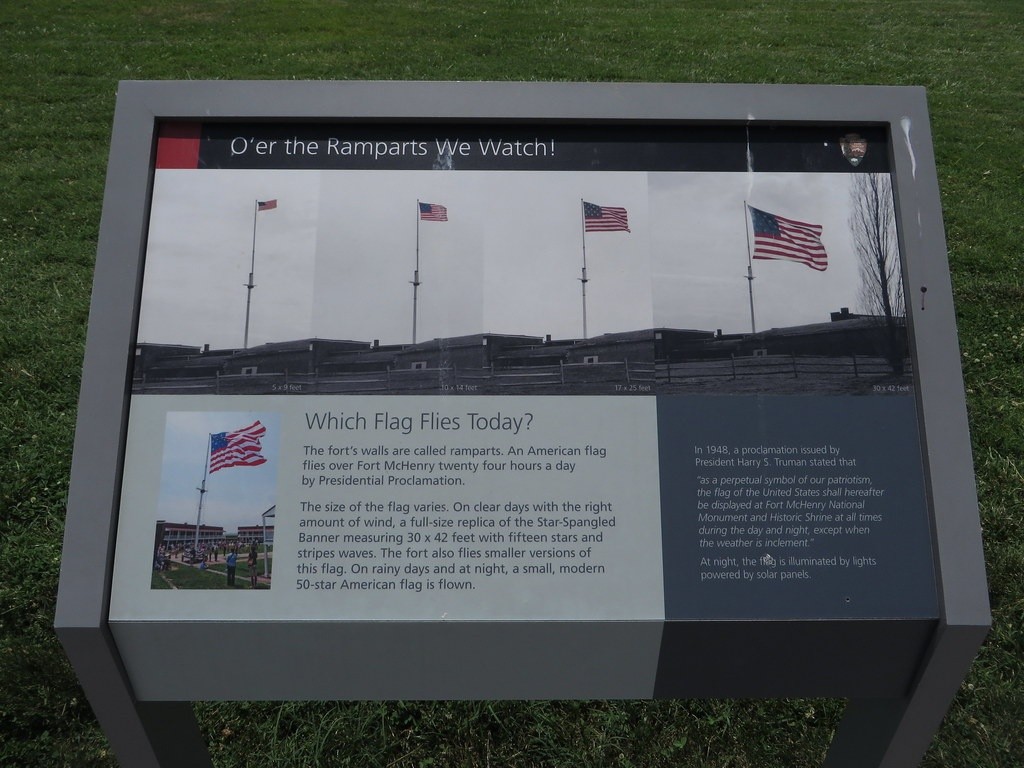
[[248, 559, 254, 566]]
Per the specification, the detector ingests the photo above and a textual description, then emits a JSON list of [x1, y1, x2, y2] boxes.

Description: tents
[[262, 505, 275, 577]]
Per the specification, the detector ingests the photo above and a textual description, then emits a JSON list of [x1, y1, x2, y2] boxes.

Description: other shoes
[[255, 586, 257, 588], [249, 586, 253, 589]]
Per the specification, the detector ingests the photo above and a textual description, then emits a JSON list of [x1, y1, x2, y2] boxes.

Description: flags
[[419, 202, 448, 221], [747, 204, 828, 271], [258, 199, 277, 211], [584, 201, 631, 233], [208, 420, 267, 474]]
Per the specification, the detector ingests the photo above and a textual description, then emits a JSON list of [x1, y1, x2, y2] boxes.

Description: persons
[[156, 539, 258, 588]]
